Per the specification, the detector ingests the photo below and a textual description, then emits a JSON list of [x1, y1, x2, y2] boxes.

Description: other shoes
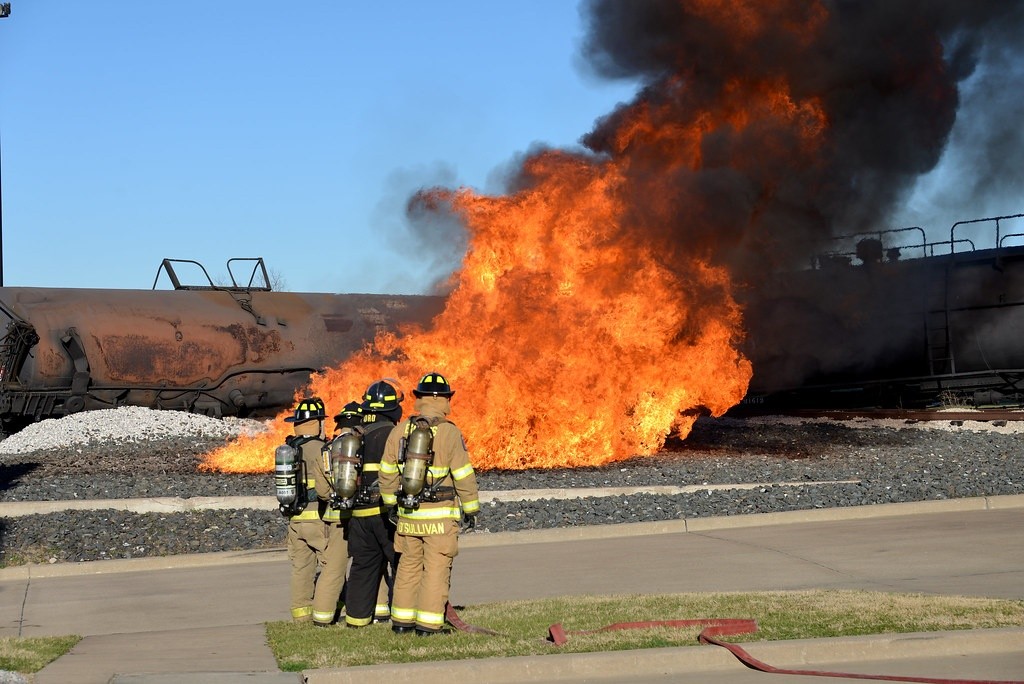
[[347, 623, 358, 629], [392, 625, 414, 634], [314, 621, 330, 627], [415, 629, 433, 637]]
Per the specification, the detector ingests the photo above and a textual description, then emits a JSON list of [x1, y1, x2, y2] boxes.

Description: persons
[[287, 378, 405, 628], [378, 373, 480, 636]]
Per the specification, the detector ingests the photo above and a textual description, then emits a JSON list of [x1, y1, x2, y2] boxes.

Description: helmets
[[334, 401, 363, 420], [284, 398, 330, 422], [360, 381, 404, 411], [413, 373, 455, 399]]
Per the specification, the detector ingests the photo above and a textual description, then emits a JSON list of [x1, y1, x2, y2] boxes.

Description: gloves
[[464, 513, 477, 533]]
